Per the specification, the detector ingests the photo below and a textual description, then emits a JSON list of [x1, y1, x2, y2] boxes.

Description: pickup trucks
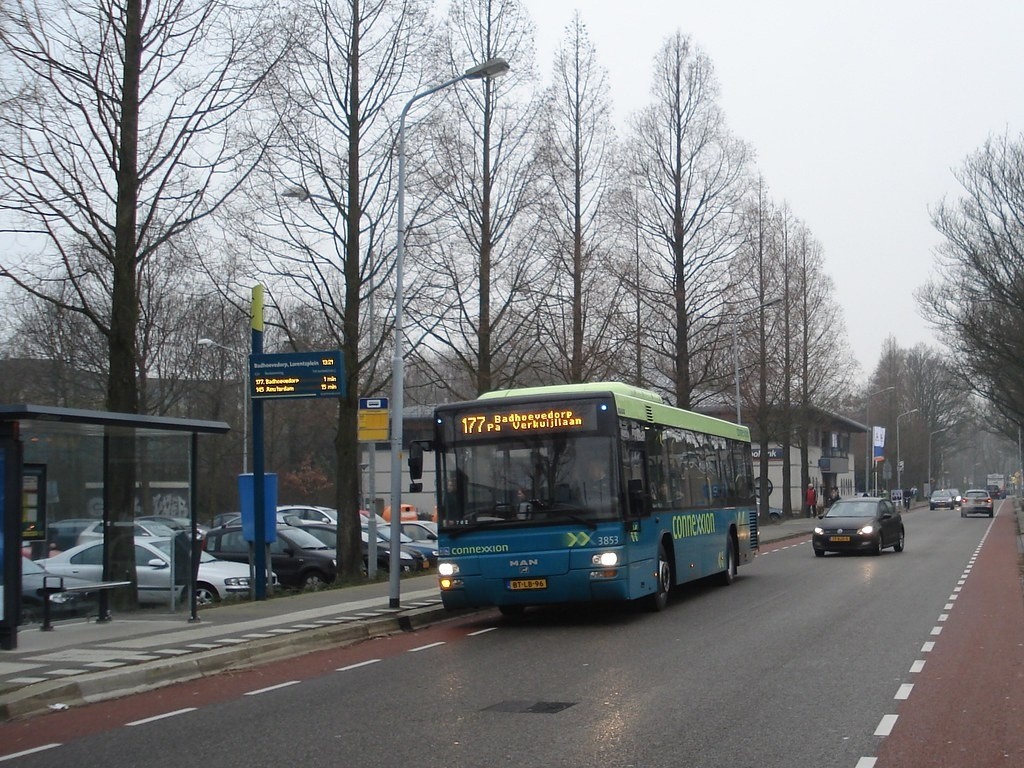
[[961, 489, 994, 518]]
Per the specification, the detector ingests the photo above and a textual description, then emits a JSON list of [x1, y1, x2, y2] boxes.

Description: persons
[[903, 484, 918, 511], [440, 457, 685, 528], [804, 483, 818, 519]]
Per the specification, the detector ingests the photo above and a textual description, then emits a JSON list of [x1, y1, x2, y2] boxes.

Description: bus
[[407, 381, 760, 616]]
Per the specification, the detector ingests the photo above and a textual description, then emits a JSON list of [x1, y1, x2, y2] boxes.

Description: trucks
[[929, 489, 956, 510], [987, 473, 1006, 499]]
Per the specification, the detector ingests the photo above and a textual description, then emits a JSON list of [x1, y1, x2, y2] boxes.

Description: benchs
[[36, 575, 131, 630]]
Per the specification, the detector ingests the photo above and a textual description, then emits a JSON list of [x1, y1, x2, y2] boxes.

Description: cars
[[947, 489, 962, 506], [290, 523, 429, 574], [383, 520, 437, 544], [376, 524, 438, 568], [360, 511, 387, 524], [812, 497, 905, 557], [210, 512, 304, 530], [134, 516, 211, 540], [21, 556, 96, 625], [201, 524, 337, 590], [276, 505, 337, 525], [48, 519, 96, 551], [985, 485, 1002, 500], [34, 537, 281, 607], [756, 501, 784, 520], [75, 520, 178, 546]]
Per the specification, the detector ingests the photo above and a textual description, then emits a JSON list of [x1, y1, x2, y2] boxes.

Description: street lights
[[896, 409, 919, 490], [928, 428, 948, 495], [733, 298, 783, 425], [865, 387, 895, 493], [281, 187, 378, 580], [389, 57, 510, 607], [198, 338, 247, 474]]
